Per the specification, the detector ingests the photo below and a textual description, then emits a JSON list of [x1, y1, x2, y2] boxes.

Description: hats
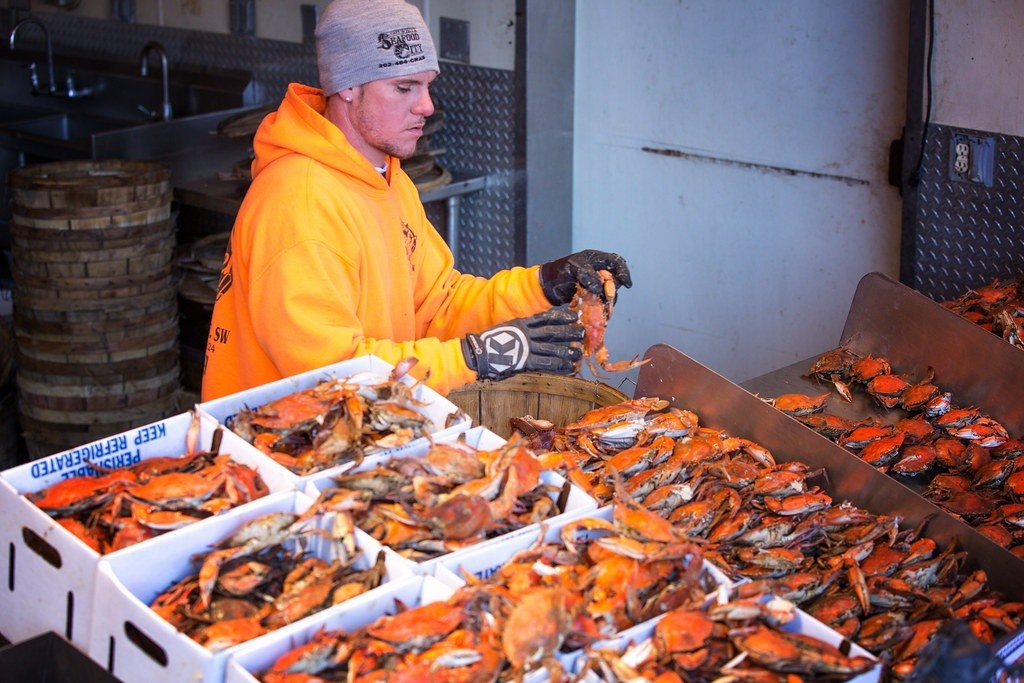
[[314, 0, 440, 96]]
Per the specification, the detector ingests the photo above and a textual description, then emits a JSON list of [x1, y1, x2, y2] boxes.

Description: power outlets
[[948, 132, 994, 188]]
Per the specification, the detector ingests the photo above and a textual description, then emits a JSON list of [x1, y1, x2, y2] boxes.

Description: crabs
[[26, 269, 1023, 683]]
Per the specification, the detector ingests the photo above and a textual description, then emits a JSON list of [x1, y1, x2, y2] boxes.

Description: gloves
[[461, 310, 585, 381], [541, 250, 631, 308]]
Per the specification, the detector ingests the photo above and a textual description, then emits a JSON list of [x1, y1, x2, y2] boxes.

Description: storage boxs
[[0, 352, 882, 682]]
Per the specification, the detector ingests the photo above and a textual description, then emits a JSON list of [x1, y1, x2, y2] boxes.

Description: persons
[[200, 0, 632, 406]]
